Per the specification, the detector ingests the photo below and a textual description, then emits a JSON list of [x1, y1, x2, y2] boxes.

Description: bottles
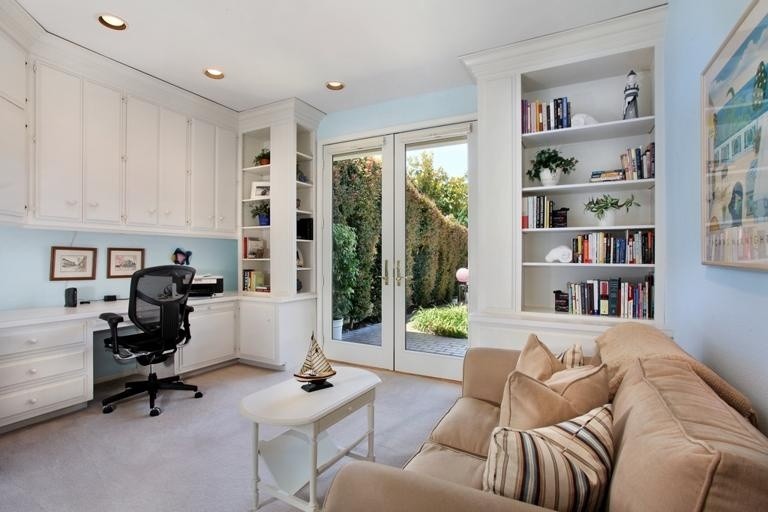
[[600, 283, 608, 315]]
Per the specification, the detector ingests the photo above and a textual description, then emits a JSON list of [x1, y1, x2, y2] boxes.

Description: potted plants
[[250, 201, 270, 226], [251, 145, 270, 166], [524, 146, 580, 186], [582, 191, 642, 227], [332, 222, 362, 340]]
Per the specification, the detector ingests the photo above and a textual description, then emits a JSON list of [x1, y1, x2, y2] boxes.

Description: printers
[[171, 274, 223, 299]]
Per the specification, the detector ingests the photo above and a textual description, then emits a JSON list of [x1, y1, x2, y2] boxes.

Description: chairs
[[98, 264, 205, 418]]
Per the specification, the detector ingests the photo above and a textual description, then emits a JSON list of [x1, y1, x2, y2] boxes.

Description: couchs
[[321, 321, 768, 511]]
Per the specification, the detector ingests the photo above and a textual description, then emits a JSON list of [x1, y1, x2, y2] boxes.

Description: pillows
[[555, 340, 585, 368], [479, 401, 617, 511], [515, 329, 566, 381], [497, 360, 611, 429]]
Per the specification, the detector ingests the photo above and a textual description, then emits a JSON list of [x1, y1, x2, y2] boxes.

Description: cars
[[711, 162, 743, 231]]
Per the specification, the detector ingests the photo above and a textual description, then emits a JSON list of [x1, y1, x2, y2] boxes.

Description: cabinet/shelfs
[[187, 118, 238, 239], [122, 90, 190, 236], [238, 117, 317, 374], [33, 58, 126, 232], [0, 30, 32, 225], [512, 43, 664, 337]]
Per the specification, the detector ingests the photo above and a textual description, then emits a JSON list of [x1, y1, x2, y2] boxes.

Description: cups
[[64, 288, 76, 308], [552, 210, 567, 227], [104, 295, 117, 301]]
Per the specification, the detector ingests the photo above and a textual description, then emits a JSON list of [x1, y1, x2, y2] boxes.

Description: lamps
[[206, 69, 224, 80]]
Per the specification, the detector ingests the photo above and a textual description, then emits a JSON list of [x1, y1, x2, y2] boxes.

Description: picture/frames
[[105, 246, 145, 279], [698, 0, 767, 273], [249, 180, 270, 200], [49, 245, 98, 282]]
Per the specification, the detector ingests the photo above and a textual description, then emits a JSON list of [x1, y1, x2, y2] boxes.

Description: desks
[[238, 364, 385, 512], [1, 292, 240, 437]]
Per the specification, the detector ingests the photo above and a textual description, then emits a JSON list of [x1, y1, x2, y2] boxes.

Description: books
[[243, 270, 270, 293], [520, 92, 654, 319]]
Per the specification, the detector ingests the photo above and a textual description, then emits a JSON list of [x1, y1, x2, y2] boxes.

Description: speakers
[[65, 287, 77, 307]]
